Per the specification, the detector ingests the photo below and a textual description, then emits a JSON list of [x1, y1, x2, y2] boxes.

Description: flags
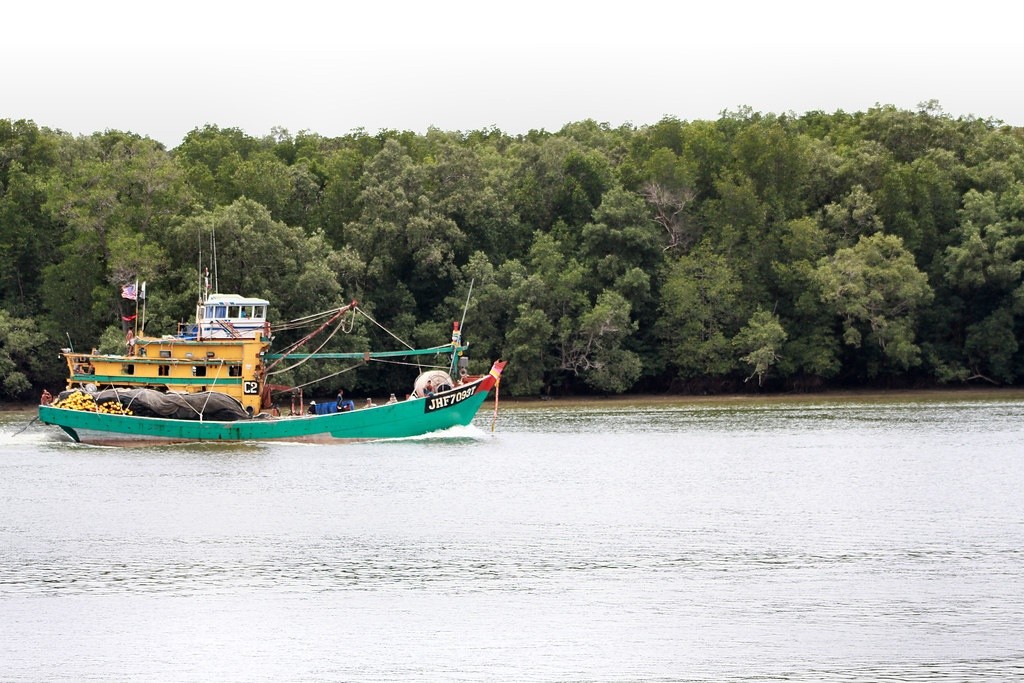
[[121, 284, 136, 300]]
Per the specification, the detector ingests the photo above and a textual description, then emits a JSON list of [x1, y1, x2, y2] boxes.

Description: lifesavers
[[42, 392, 52, 402]]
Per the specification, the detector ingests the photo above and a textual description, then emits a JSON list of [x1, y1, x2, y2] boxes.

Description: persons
[[125, 326, 135, 355], [459, 366, 468, 383], [452, 322, 463, 356], [41, 389, 52, 405], [273, 380, 433, 415]]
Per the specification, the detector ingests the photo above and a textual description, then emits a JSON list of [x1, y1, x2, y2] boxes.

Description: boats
[[38, 218, 509, 443]]
